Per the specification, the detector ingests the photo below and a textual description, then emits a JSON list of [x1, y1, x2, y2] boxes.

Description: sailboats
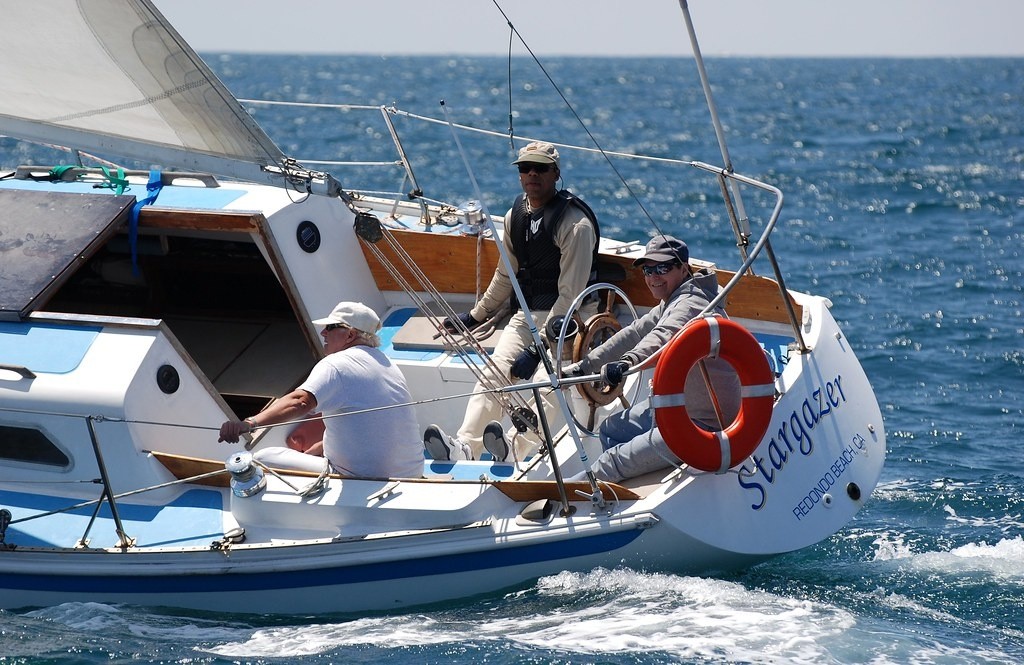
[[0, 0, 887, 621]]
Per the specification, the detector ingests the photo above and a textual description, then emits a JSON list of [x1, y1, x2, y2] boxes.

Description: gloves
[[510, 349, 541, 380], [601, 362, 629, 387], [555, 363, 584, 390]]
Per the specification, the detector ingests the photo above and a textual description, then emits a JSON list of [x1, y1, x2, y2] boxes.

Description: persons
[[217, 301, 424, 479], [561, 235, 741, 483], [423, 140, 601, 460]]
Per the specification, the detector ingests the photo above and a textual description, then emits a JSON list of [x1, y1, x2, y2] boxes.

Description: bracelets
[[245, 416, 257, 433]]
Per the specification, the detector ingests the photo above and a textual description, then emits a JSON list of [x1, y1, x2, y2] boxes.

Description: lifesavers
[[653, 316, 777, 473]]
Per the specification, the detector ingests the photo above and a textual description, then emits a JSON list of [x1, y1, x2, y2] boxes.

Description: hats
[[313, 302, 382, 333], [634, 235, 689, 263], [511, 142, 560, 166]]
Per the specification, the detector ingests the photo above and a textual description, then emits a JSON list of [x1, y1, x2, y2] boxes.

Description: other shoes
[[484, 420, 513, 464], [424, 424, 472, 462]]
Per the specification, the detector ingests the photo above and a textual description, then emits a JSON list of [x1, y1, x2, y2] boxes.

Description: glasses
[[642, 261, 679, 277], [518, 163, 555, 173], [325, 323, 350, 332]]
